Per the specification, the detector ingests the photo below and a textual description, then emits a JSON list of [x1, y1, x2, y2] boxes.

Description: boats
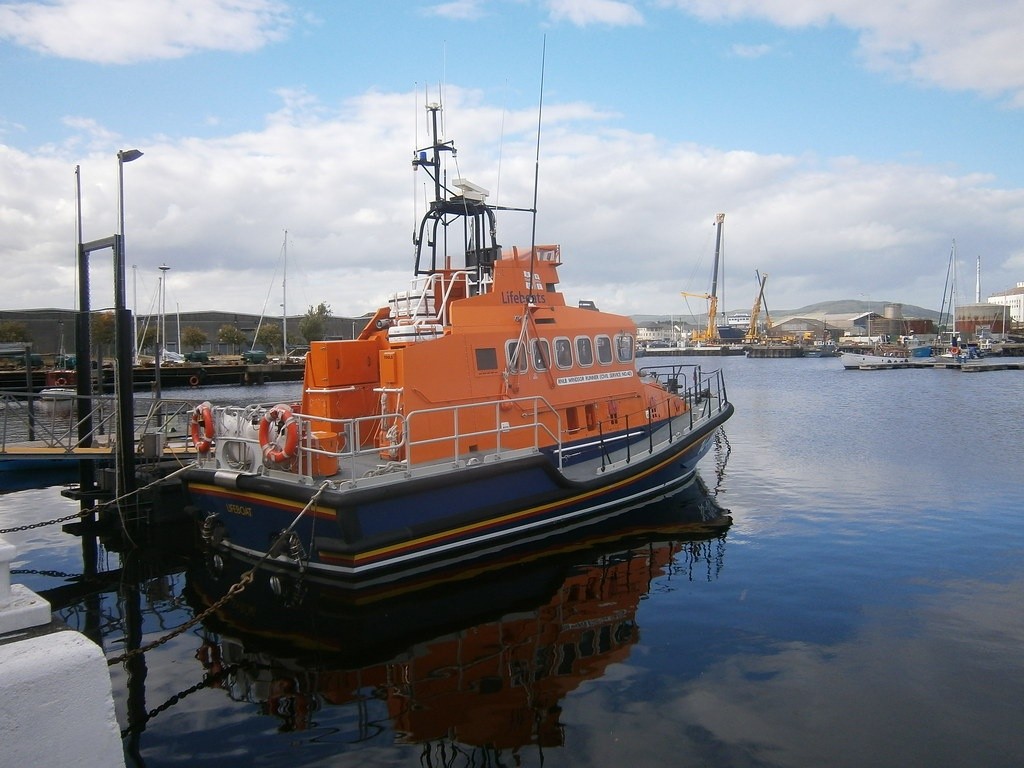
[[170, 32, 736, 611], [841, 351, 936, 369], [177, 466, 733, 767]]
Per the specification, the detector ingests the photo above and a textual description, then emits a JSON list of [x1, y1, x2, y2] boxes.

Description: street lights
[[118, 148, 143, 308], [353, 322, 358, 340], [160, 263, 172, 351]]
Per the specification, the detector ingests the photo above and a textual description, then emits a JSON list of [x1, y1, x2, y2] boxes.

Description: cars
[[958, 339, 995, 360]]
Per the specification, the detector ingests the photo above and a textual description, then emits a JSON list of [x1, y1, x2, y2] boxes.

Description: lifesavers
[[191, 405, 213, 452], [258, 407, 296, 462], [951, 347, 959, 354]]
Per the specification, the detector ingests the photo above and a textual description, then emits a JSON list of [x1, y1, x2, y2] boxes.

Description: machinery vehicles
[[680, 213, 726, 342], [743, 270, 815, 345]]
